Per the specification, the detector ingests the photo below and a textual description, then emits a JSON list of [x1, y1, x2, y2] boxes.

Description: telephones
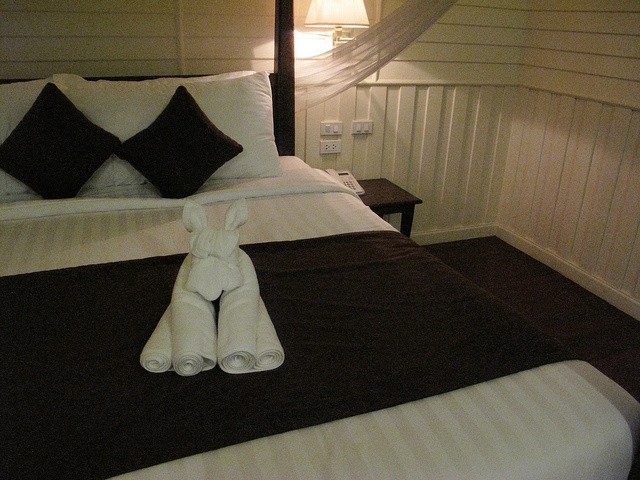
[[324, 169, 365, 196]]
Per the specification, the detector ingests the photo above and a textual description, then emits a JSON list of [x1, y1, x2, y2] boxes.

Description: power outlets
[[320, 141, 343, 154]]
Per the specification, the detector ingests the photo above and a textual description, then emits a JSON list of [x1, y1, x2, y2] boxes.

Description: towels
[[176, 199, 248, 299], [136, 250, 288, 376]]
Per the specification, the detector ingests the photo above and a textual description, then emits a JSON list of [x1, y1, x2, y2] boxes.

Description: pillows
[[1, 81, 119, 198], [52, 70, 283, 181], [111, 85, 245, 199]]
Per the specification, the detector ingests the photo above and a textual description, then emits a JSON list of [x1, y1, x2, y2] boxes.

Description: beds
[[1, 63, 638, 478]]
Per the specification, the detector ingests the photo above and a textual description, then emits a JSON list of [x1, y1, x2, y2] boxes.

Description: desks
[[353, 177, 424, 242]]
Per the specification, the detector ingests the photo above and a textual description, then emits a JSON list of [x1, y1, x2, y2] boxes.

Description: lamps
[[302, 0, 371, 31]]
